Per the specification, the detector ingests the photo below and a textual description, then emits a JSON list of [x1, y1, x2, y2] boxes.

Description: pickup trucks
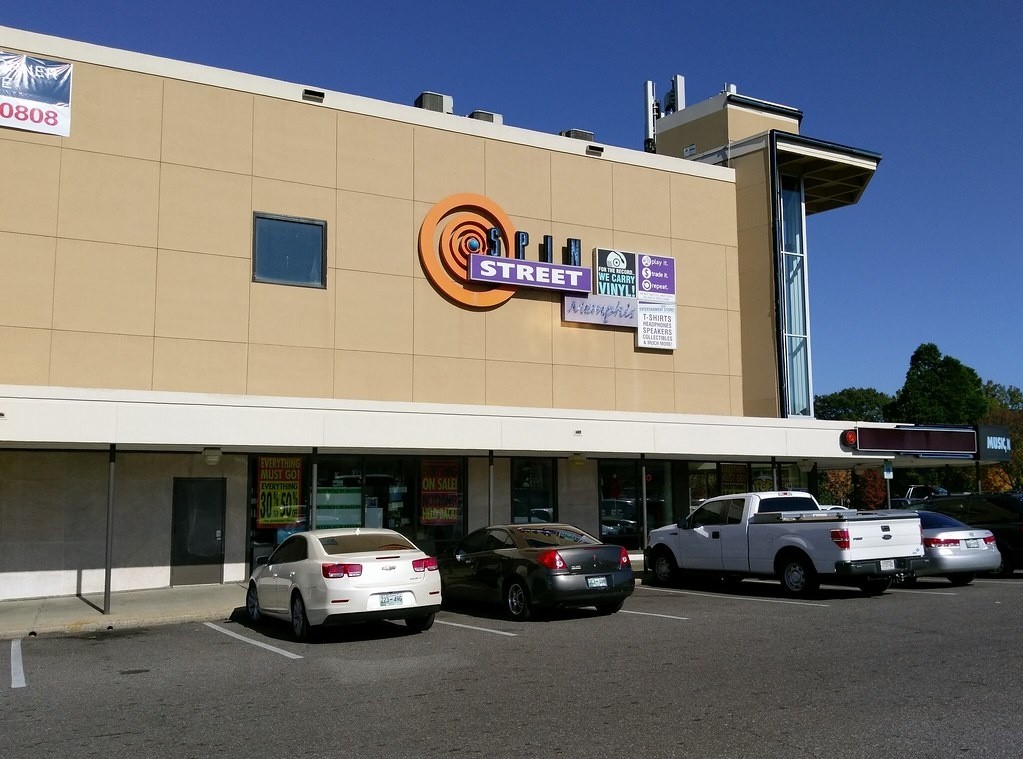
[[643, 491, 925, 599]]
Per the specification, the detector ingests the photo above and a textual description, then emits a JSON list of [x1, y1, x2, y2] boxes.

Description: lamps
[[568, 455, 586, 466], [202, 449, 222, 466]]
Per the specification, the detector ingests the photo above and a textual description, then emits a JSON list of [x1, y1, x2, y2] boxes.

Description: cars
[[511, 499, 707, 546], [434, 524, 636, 621], [878, 484, 1023, 588], [247, 527, 443, 642]]
